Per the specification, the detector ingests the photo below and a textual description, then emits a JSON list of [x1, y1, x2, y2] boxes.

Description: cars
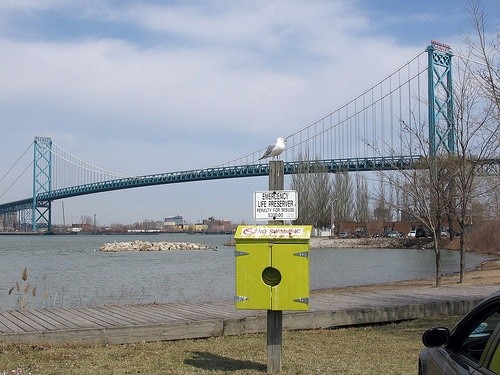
[[387, 231, 401, 237], [372, 231, 388, 238], [440, 229, 448, 237], [406, 231, 416, 238], [418, 290, 500, 375], [339, 232, 365, 238]]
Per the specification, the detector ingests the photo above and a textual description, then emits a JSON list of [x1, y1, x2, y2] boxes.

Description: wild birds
[[257, 137, 287, 161]]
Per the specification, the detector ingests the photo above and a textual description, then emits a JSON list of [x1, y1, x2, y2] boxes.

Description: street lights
[[329, 199, 337, 238]]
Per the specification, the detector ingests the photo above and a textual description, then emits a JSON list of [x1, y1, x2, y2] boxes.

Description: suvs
[[415, 228, 428, 238]]
